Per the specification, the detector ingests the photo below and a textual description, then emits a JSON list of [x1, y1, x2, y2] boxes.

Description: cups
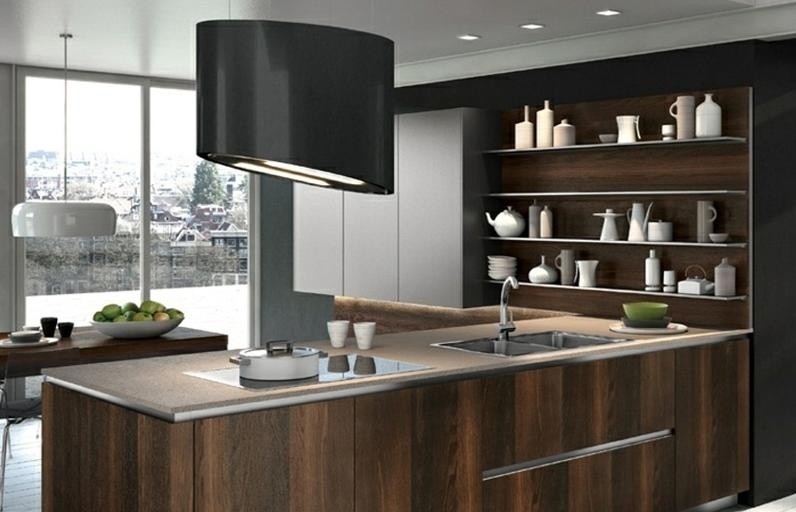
[[696, 200, 717, 242], [41, 317, 58, 336], [58, 322, 73, 339], [575, 260, 600, 287], [353, 322, 377, 350], [327, 320, 351, 348], [669, 96, 695, 140], [615, 115, 642, 143], [555, 249, 575, 285]]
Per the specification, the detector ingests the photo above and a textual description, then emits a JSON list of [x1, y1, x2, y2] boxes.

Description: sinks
[[430, 338, 560, 357], [508, 331, 634, 348]]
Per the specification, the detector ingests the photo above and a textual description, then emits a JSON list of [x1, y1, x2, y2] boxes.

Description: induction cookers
[[182, 354, 432, 392]]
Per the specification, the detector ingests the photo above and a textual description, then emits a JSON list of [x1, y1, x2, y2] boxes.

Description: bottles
[[645, 249, 662, 292], [663, 269, 676, 293], [661, 124, 674, 140], [528, 199, 553, 238]]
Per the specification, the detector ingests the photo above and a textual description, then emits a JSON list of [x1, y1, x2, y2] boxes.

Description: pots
[[229, 340, 327, 381]]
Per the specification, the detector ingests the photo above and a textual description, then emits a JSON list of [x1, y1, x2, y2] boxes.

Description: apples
[[93, 300, 185, 323]]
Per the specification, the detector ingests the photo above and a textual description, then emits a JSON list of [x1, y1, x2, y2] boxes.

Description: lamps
[[11, 23, 116, 238]]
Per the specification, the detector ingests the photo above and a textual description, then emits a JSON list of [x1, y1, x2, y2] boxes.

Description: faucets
[[496, 276, 519, 340]]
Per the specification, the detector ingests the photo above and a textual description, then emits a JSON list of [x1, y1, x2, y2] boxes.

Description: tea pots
[[627, 201, 653, 241], [684, 263, 714, 295], [485, 206, 526, 237]]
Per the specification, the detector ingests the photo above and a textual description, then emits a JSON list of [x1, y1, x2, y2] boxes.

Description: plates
[[621, 318, 673, 328], [90, 318, 184, 339], [0, 337, 59, 349], [487, 254, 517, 281]]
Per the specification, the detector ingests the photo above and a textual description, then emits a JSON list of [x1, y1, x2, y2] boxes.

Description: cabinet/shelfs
[[290, 178, 343, 298], [480, 86, 753, 327], [342, 113, 398, 303], [398, 107, 503, 308]]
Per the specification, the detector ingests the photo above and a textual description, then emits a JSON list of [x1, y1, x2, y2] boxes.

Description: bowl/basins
[[598, 134, 617, 142], [622, 302, 669, 318], [709, 233, 729, 243]]
